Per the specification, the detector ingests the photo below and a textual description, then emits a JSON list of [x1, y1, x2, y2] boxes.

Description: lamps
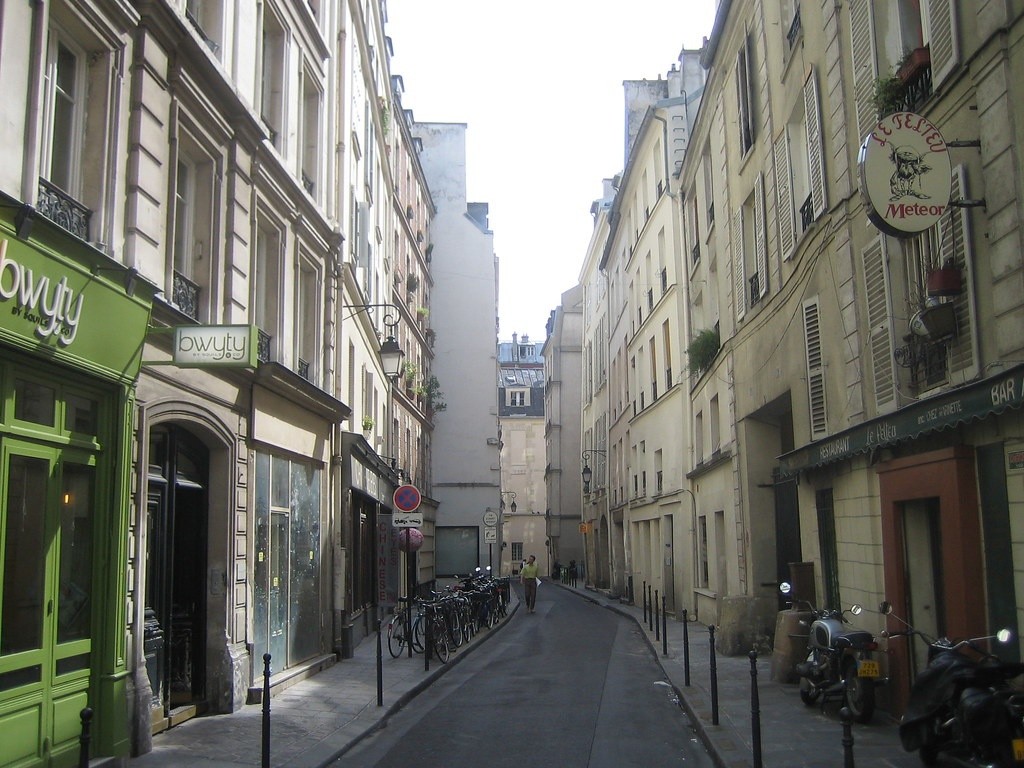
[[582, 450, 607, 484], [377, 462, 411, 485], [365, 450, 396, 469], [93, 264, 138, 297], [0, 203, 35, 241], [501, 491, 517, 512]]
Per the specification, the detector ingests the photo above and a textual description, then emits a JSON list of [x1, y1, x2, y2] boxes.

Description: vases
[[920, 302, 955, 340], [909, 309, 931, 340], [927, 268, 962, 295]]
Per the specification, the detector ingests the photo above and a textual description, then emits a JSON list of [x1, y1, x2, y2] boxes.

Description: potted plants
[[406, 386, 426, 402], [362, 415, 375, 440], [871, 45, 930, 120], [405, 360, 417, 387], [417, 307, 429, 321]]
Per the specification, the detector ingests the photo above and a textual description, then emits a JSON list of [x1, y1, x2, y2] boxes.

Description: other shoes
[[530, 608, 536, 613]]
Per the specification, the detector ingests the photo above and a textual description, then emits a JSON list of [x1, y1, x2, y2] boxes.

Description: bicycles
[[387, 566, 507, 665]]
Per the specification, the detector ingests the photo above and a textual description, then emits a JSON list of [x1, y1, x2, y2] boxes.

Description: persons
[[520, 554, 539, 613]]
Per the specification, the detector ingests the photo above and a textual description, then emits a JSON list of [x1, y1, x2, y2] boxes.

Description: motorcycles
[[778, 583, 889, 722], [878, 602, 1024, 768]]
